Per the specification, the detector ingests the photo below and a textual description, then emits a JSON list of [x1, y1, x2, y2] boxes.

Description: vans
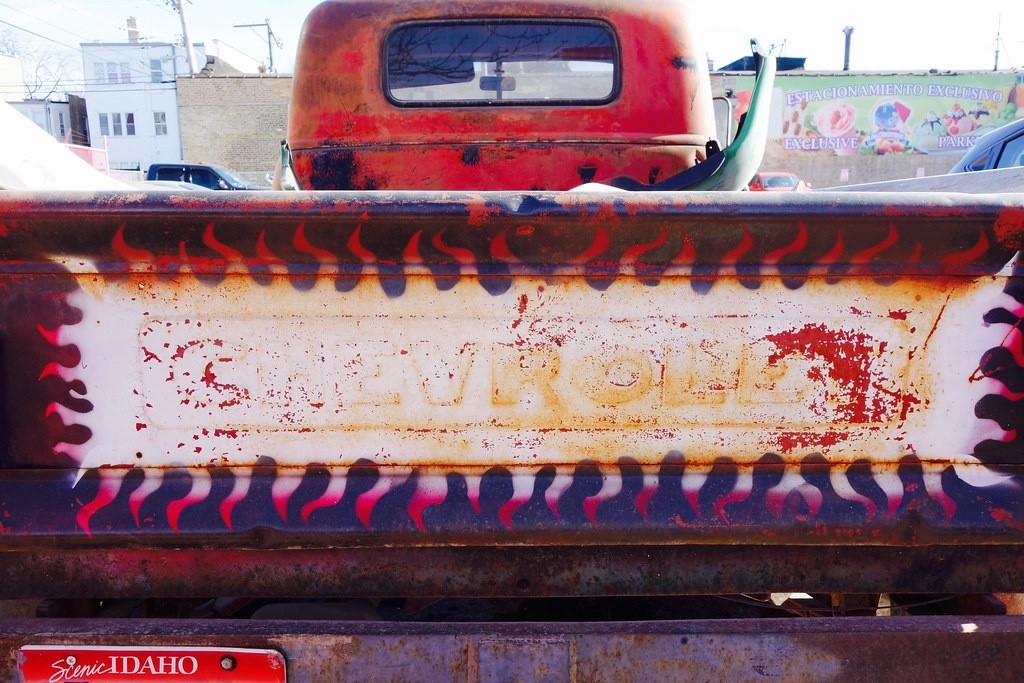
[[281, 1, 723, 191]]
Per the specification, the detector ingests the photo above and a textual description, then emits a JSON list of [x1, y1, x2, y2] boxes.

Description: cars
[[949, 115, 1024, 174], [748, 172, 811, 192], [143, 160, 270, 192]]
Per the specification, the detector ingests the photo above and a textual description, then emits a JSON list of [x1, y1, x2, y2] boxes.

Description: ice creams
[[917, 103, 972, 149]]
